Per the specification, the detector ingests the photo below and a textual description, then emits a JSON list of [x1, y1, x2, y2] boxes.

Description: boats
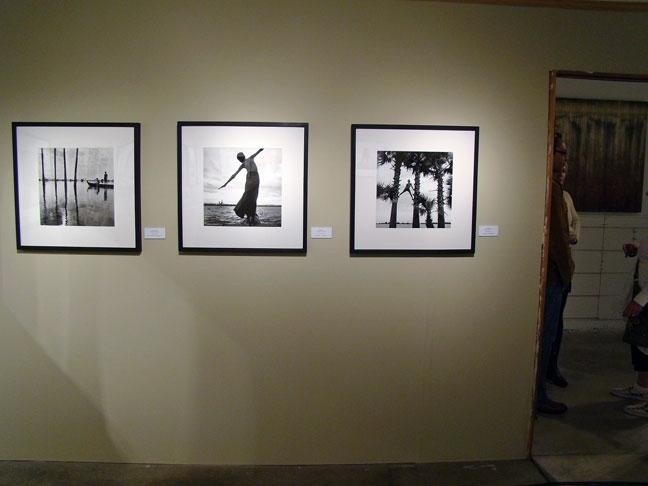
[[85, 180, 113, 188]]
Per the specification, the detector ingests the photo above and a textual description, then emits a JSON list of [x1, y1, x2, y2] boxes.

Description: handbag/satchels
[[622, 281, 648, 347]]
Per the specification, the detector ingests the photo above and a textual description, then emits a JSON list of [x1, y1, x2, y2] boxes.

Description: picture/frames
[[177, 121, 309, 256], [350, 123, 480, 258], [12, 121, 142, 256]]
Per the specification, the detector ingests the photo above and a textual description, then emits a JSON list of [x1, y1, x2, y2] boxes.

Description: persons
[[104, 172, 108, 181], [96, 178, 101, 184], [610, 237, 648, 418], [218, 148, 263, 225], [534, 130, 571, 414], [399, 178, 414, 200], [552, 161, 580, 386]]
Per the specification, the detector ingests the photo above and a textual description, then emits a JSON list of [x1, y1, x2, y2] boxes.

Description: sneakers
[[624, 402, 648, 416], [609, 383, 648, 400], [537, 399, 568, 415], [547, 368, 568, 388]]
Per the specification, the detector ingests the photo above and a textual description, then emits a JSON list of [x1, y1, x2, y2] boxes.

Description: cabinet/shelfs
[[562, 215, 634, 331]]
